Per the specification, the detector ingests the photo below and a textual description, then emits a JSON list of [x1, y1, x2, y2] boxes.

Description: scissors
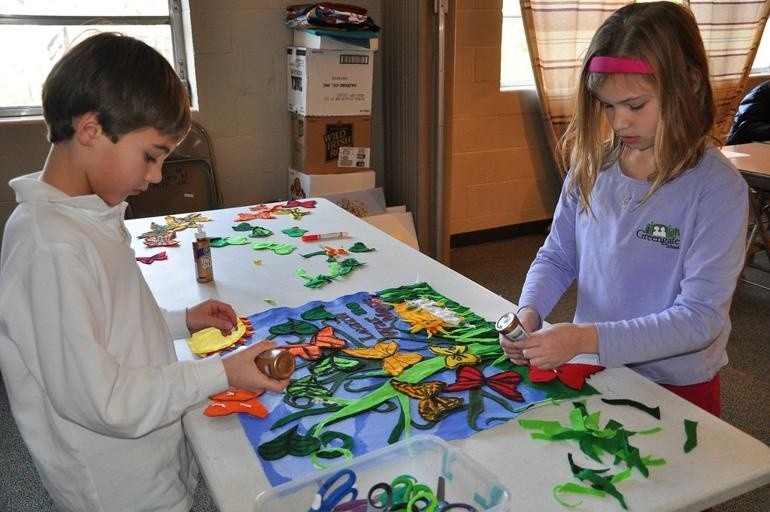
[[318, 243, 347, 256], [307, 469, 476, 512]]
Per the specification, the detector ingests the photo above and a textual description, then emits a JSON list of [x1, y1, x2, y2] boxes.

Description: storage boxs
[[288, 168, 376, 198], [286, 46, 374, 116], [291, 113, 373, 174], [293, 29, 381, 52]]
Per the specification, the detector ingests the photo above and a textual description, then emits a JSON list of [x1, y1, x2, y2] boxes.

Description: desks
[[715, 142, 770, 292], [122, 196, 770, 512]]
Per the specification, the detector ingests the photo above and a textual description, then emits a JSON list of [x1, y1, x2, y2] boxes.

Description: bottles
[[192, 225, 213, 283], [236, 345, 296, 380], [495, 312, 557, 373]]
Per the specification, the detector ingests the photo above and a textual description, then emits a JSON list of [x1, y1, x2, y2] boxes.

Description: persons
[[497, 2, 752, 419], [0, 29, 293, 511]]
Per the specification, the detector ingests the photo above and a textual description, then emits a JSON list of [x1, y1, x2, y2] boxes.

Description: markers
[[302, 232, 348, 241]]
